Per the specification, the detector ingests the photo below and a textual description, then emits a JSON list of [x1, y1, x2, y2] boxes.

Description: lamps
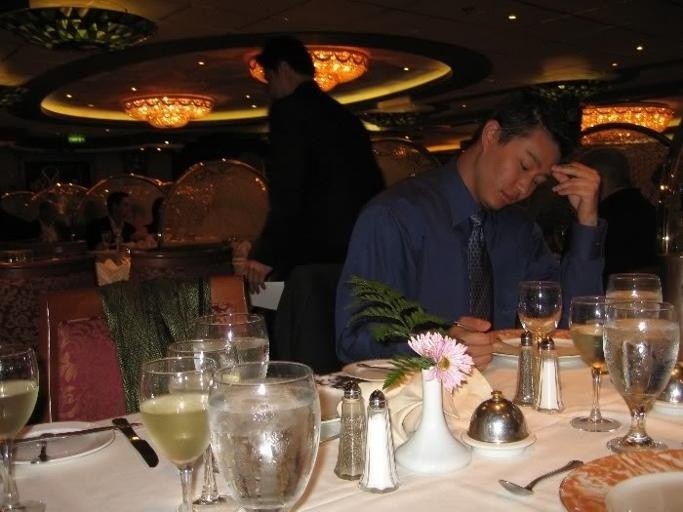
[[242, 39, 372, 95], [534, 78, 607, 103], [0, 4, 161, 53], [120, 88, 217, 131], [355, 109, 434, 130], [575, 102, 674, 143]]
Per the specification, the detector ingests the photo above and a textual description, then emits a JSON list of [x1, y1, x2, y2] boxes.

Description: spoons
[[500, 458, 582, 497], [29, 433, 54, 466]]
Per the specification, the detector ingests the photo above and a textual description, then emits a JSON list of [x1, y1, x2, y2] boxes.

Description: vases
[[393, 367, 469, 476]]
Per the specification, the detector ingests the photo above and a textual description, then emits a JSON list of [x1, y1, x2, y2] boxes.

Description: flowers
[[337, 268, 477, 397]]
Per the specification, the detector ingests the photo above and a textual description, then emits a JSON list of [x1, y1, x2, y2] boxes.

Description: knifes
[[9, 422, 139, 447], [113, 418, 161, 471]]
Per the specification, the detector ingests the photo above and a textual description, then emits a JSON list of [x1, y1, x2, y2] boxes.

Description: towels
[[93, 254, 131, 288]]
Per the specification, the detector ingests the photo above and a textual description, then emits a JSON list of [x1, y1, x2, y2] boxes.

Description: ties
[[462, 212, 493, 323]]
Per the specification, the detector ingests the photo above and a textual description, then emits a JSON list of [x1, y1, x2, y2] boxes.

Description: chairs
[[38, 273, 265, 429], [116, 241, 233, 284], [0, 241, 90, 426]]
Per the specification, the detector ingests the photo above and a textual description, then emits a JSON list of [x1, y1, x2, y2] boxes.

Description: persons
[[28, 200, 70, 242], [245, 38, 385, 377], [335, 95, 606, 373], [87, 190, 136, 248], [577, 147, 665, 295], [135, 196, 165, 240]]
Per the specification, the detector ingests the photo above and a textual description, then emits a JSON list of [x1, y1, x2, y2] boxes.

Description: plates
[[344, 359, 402, 382], [489, 329, 583, 359], [559, 447, 683, 512], [7, 422, 116, 464]]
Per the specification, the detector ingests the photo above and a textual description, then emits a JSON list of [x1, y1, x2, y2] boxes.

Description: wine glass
[[513, 270, 682, 459], [139, 309, 271, 512], [0, 342, 44, 512]]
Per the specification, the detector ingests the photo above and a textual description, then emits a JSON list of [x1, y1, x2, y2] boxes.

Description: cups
[[205, 360, 320, 511]]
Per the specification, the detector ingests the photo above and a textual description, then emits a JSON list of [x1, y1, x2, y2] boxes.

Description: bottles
[[361, 393, 399, 494], [332, 383, 367, 483]]
